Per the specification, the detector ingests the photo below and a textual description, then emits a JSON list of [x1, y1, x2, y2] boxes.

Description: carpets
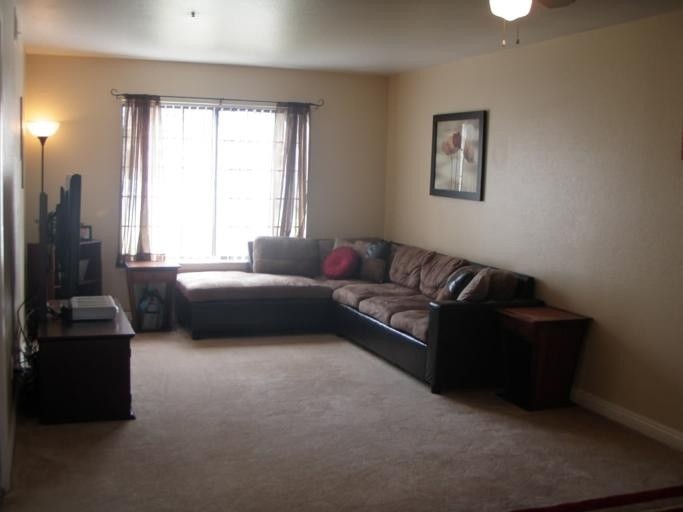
[[508, 483, 683, 512]]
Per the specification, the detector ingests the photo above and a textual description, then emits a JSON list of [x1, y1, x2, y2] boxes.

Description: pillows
[[250, 235, 518, 303]]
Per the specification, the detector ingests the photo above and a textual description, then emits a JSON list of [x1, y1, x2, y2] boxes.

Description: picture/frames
[[80, 225, 92, 241], [429, 110, 487, 201]]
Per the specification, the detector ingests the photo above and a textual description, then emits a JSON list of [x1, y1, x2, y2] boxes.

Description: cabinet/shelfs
[[26, 295, 136, 424], [26, 240, 102, 344]]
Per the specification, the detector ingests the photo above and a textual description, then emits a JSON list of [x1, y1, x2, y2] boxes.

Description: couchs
[[175, 261, 546, 394]]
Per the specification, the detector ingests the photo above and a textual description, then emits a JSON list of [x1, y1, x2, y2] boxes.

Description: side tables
[[493, 305, 594, 412], [124, 261, 182, 333]]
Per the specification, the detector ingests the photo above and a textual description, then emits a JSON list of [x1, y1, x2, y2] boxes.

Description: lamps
[[488, 0, 533, 47], [27, 119, 60, 242]]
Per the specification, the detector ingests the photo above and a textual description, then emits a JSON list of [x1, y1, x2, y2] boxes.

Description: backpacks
[[138, 287, 166, 330]]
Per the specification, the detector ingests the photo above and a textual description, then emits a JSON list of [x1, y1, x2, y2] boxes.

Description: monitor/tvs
[[56, 173, 81, 295]]
